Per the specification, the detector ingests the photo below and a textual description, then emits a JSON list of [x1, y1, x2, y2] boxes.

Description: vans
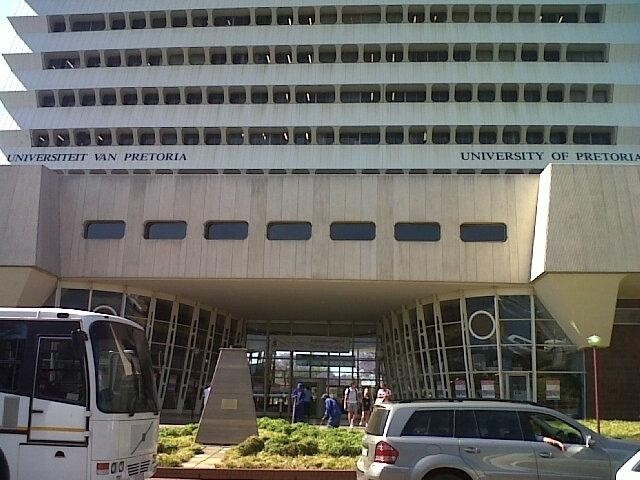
[[356, 400, 640, 480]]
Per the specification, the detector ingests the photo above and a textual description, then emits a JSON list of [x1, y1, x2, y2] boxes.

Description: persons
[[291, 382, 305, 421], [320, 393, 341, 428], [377, 382, 392, 399], [304, 385, 313, 417], [358, 387, 371, 427], [343, 381, 360, 428]]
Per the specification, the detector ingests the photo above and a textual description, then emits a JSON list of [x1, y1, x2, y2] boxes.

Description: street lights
[[585, 334, 603, 434]]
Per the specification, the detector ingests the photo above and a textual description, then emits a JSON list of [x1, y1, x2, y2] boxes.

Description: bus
[[0, 305, 162, 480]]
